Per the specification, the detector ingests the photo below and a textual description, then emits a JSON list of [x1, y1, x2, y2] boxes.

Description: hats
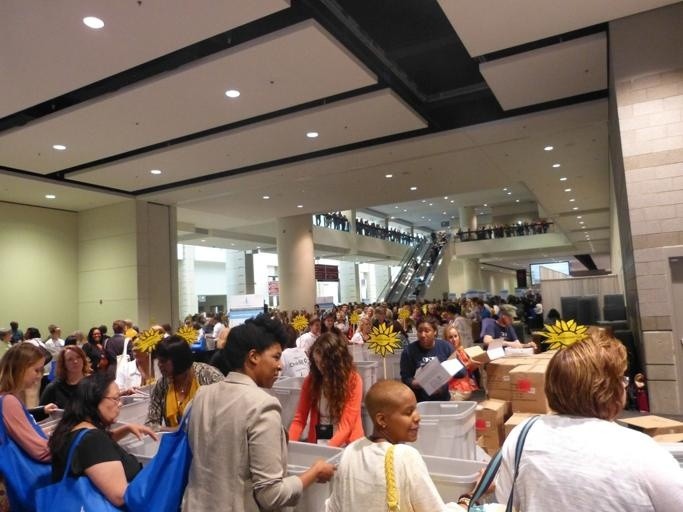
[[500, 304, 517, 318]]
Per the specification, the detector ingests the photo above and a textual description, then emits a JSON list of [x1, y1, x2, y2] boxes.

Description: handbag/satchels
[[116, 354, 131, 368], [0, 394, 189, 512], [97, 354, 109, 370]]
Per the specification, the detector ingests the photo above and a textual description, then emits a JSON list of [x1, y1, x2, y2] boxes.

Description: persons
[[315, 210, 550, 271], [1, 279, 683, 510]]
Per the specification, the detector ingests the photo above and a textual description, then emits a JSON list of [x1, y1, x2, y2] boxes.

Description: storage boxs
[[29, 344, 683, 511]]
